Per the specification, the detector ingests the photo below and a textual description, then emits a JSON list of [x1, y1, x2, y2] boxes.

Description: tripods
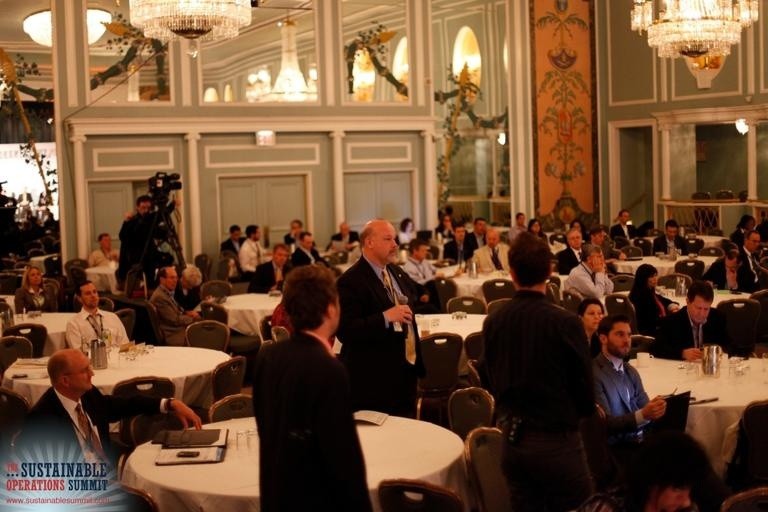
[[129, 207, 187, 296]]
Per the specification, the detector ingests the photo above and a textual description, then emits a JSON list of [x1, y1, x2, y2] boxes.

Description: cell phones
[[177, 452, 199, 457]]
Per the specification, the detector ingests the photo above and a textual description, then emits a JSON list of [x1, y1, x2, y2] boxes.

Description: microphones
[[398, 296, 408, 338]]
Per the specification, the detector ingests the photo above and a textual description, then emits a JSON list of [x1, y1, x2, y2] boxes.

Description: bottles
[[81, 337, 89, 357]]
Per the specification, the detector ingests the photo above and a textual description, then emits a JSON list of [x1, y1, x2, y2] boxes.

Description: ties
[[90, 313, 104, 340], [492, 247, 501, 269], [75, 404, 105, 463], [382, 266, 417, 367], [591, 272, 596, 284]]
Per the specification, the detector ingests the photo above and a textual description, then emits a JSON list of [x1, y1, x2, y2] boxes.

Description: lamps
[[126, 0, 254, 59], [626, 0, 763, 76]]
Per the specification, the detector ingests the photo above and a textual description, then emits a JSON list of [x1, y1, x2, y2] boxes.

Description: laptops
[[416, 230, 432, 242], [632, 391, 690, 456]]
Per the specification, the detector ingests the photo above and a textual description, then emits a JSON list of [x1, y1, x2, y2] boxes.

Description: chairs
[[376, 468, 467, 512], [378, 186, 768, 361], [413, 329, 464, 427], [448, 377, 496, 444], [465, 352, 480, 386], [0, 213, 376, 458], [7, 427, 159, 512], [718, 488, 768, 512], [462, 420, 514, 512], [464, 329, 488, 358]]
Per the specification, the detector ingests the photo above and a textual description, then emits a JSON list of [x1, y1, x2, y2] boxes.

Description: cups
[[688, 253, 693, 259], [269, 291, 281, 303], [667, 291, 673, 299], [102, 329, 111, 352], [636, 352, 654, 368], [119, 344, 153, 363], [728, 358, 747, 379], [669, 246, 677, 262], [431, 318, 441, 331], [420, 319, 430, 338], [684, 359, 699, 380], [762, 352, 768, 383], [235, 427, 259, 455], [27, 311, 43, 322]]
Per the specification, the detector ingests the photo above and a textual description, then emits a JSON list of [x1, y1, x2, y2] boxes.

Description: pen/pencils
[[672, 387, 678, 396]]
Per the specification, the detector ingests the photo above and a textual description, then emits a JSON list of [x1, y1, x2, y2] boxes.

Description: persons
[[2, 180, 767, 512]]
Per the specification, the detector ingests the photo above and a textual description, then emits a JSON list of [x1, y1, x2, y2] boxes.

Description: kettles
[[675, 276, 686, 297], [91, 339, 108, 369], [702, 343, 723, 377], [0, 303, 15, 336]]
